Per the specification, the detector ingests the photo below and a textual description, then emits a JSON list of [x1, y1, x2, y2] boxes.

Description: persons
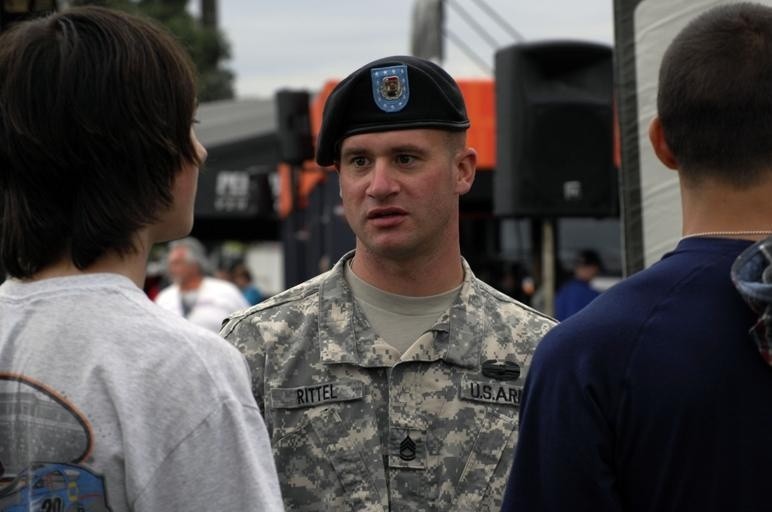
[[237, 271, 267, 306], [552, 246, 605, 323], [152, 235, 253, 336], [0, 7, 286, 512], [501, 3, 771, 509], [213, 53, 565, 511], [214, 241, 247, 286]]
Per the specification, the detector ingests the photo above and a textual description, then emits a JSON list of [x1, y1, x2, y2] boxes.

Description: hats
[[316, 56, 471, 167]]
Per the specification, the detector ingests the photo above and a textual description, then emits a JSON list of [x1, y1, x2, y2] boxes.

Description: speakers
[[278, 92, 315, 164], [495, 40, 621, 218]]
